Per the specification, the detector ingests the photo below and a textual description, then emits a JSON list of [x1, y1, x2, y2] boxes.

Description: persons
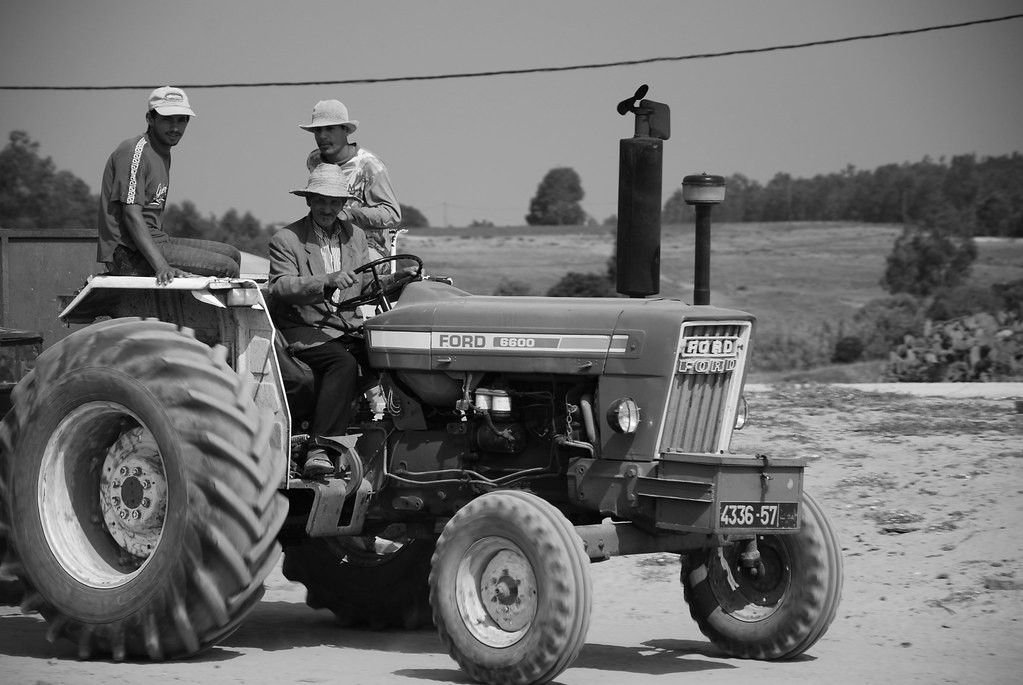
[[298, 100, 402, 275], [267, 162, 425, 473], [96, 85, 242, 285]]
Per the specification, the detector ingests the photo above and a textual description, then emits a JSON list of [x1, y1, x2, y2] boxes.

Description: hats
[[299, 100, 359, 134], [148, 86, 197, 117], [289, 163, 364, 204]]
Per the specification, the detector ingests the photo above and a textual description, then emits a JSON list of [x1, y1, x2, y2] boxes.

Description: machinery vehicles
[[0, 81, 845, 685]]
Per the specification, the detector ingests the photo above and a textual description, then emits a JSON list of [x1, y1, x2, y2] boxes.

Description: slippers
[[304, 449, 335, 473]]
